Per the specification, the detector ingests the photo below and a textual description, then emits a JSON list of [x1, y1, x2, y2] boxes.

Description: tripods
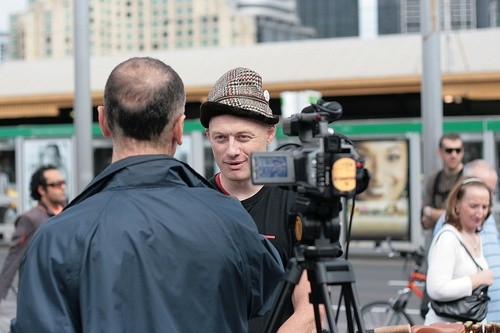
[[262, 196, 365, 333]]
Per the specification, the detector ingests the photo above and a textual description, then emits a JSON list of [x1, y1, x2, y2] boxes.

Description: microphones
[[301, 102, 344, 125]]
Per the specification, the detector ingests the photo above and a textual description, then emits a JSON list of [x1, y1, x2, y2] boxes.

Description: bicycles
[[353, 236, 427, 333]]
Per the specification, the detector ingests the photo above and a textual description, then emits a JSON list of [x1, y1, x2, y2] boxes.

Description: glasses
[[444, 148, 462, 154], [45, 180, 68, 188]]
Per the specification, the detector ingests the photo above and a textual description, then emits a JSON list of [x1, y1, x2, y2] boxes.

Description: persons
[[421, 176, 493, 326], [421, 132, 466, 255], [433, 159, 500, 325], [7, 57, 287, 333], [198, 67, 331, 333], [0, 164, 69, 300], [346, 141, 410, 237]]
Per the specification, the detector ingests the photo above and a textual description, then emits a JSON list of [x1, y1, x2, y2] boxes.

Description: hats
[[199, 67, 280, 128]]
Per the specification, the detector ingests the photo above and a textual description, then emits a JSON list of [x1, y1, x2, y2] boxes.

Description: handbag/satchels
[[430, 284, 492, 323]]
